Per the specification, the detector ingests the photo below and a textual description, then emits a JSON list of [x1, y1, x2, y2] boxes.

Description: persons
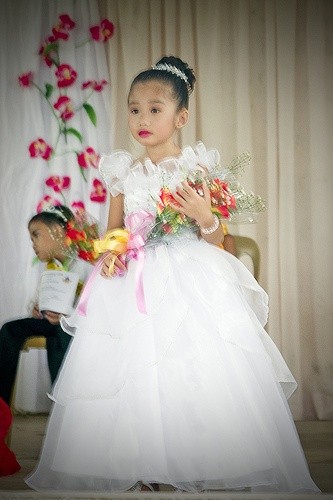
[[0, 203, 96, 407], [26, 55, 320, 491]]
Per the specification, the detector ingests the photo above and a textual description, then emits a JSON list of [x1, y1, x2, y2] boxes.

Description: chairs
[[13, 337, 54, 414]]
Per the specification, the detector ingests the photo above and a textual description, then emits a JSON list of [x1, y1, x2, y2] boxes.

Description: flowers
[[43, 202, 104, 265], [17, 12, 116, 214], [140, 150, 267, 251]]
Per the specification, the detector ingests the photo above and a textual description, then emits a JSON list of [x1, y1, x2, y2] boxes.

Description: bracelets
[[200, 214, 220, 234]]
